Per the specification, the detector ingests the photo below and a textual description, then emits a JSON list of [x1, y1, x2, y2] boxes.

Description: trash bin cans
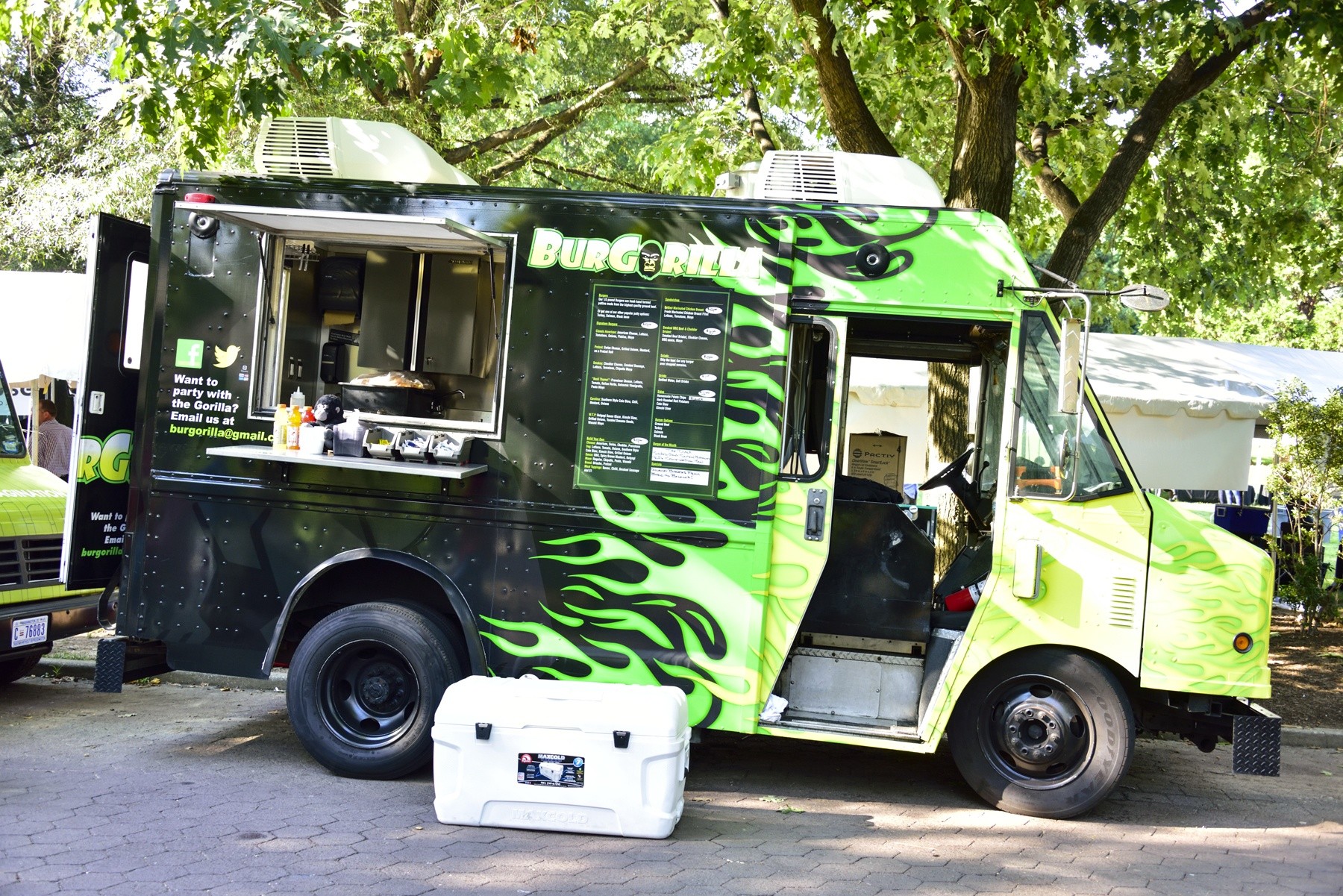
[[1232, 485, 1254, 506], [1218, 490, 1226, 504], [1269, 504, 1289, 536], [1337, 508, 1343, 543], [1223, 490, 1235, 505], [1311, 509, 1334, 542]]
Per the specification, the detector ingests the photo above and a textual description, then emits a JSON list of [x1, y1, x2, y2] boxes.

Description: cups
[[290, 386, 306, 410]]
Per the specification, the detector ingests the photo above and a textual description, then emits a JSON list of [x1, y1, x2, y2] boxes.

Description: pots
[[336, 381, 466, 419]]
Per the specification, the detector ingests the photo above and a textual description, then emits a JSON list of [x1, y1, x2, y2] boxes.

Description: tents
[[0, 271, 1343, 522]]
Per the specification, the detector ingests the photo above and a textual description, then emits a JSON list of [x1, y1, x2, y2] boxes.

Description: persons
[[27, 399, 73, 483]]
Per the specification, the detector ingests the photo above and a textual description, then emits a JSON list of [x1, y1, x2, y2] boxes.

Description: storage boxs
[[338, 382, 445, 418], [430, 675, 692, 839]]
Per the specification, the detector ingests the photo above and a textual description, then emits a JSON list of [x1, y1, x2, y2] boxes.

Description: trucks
[[59, 114, 1280, 823], [0, 359, 120, 686]]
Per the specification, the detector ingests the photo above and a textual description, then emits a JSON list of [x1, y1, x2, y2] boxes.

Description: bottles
[[272, 404, 287, 449], [301, 406, 316, 422], [287, 405, 302, 450]]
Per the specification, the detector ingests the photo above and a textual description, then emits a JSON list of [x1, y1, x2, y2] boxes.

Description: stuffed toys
[[301, 395, 345, 455]]
